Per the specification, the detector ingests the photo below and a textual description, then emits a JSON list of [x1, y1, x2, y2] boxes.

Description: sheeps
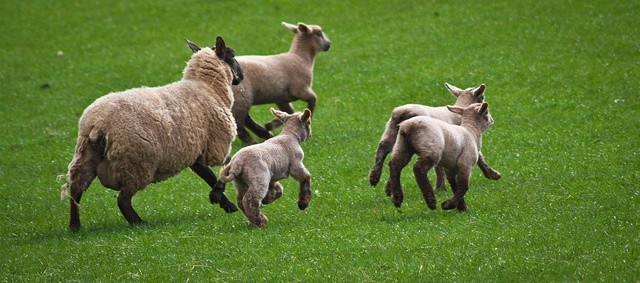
[[369, 82, 501, 196], [219, 107, 312, 230], [389, 102, 494, 214], [230, 22, 331, 145], [57, 36, 244, 233]]
